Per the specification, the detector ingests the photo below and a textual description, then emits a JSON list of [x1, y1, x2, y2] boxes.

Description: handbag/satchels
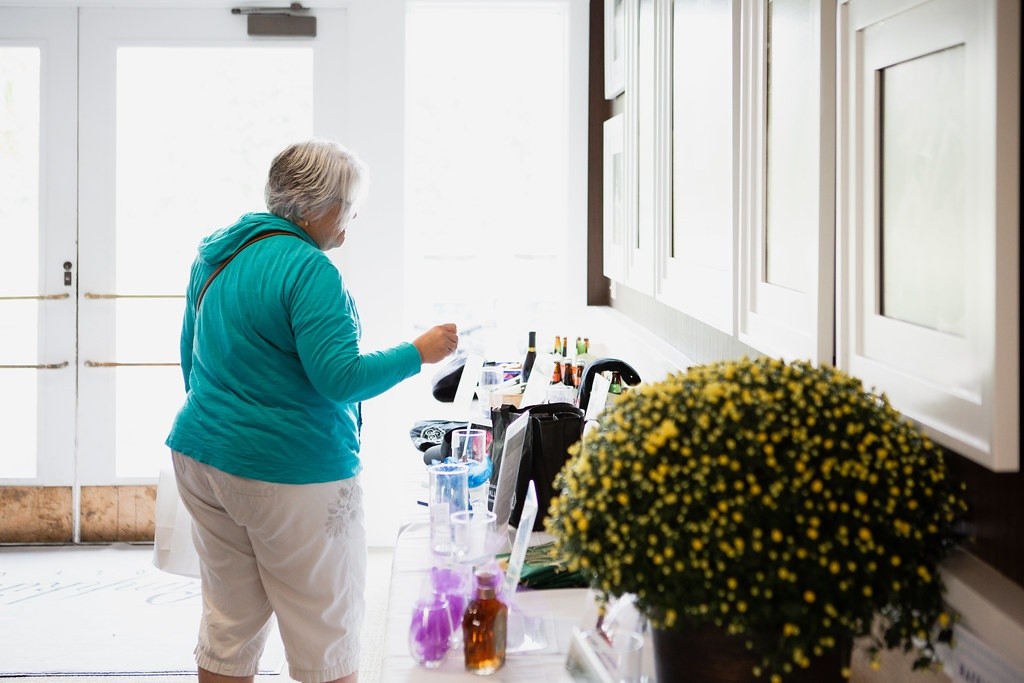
[[487, 400, 584, 532], [152, 466, 201, 578]]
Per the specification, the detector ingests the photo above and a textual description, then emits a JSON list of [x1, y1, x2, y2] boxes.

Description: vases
[[653, 614, 857, 683]]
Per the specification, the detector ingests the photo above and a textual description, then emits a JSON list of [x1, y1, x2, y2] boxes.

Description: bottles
[[461, 571, 509, 677], [549, 336, 590, 390], [603, 371, 629, 412], [520, 331, 537, 393]]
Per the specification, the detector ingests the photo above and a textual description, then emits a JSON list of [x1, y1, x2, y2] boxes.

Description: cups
[[452, 509, 497, 566], [428, 464, 469, 557], [451, 429, 486, 467], [479, 367, 503, 419]]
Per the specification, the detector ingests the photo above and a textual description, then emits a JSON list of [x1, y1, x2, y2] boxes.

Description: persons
[[163, 141, 458, 683]]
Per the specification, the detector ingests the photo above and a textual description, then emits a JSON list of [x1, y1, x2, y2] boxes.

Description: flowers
[[543, 355, 968, 681]]
